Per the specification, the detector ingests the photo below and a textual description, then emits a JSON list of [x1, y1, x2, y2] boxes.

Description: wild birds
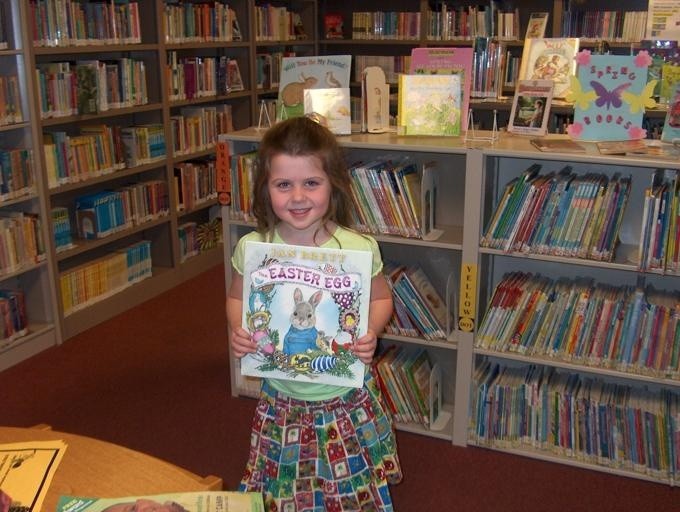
[[325, 70, 342, 88]]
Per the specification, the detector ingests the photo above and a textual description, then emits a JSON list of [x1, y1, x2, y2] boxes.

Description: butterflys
[[588, 79, 633, 112]]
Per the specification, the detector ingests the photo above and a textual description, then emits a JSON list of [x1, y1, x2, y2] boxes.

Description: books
[[275, 1, 679, 489], [240, 242, 373, 388], [0, 2, 304, 343]]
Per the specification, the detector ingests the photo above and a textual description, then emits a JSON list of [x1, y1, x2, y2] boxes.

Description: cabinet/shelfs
[[213, 125, 679, 487], [316, 1, 680, 140], [1, 2, 315, 385]]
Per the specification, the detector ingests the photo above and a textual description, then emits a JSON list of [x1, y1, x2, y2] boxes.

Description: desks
[[0, 427, 222, 512]]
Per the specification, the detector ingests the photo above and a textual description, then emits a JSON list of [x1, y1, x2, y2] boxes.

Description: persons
[[227, 117, 403, 511]]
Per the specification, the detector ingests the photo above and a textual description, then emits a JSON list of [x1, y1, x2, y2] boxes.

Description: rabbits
[[280, 72, 319, 108]]
